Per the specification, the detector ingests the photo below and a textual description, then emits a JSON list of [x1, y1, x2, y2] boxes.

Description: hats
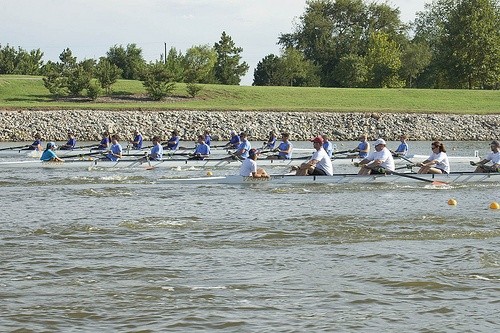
[[47, 142, 56, 147], [311, 137, 324, 143], [373, 139, 386, 145]]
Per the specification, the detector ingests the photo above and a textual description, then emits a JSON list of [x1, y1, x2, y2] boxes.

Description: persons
[[204, 130, 210, 146], [240, 148, 270, 178], [470, 139, 500, 173], [91, 131, 109, 150], [130, 130, 142, 150], [40, 142, 62, 161], [346, 135, 369, 158], [392, 134, 408, 157], [266, 133, 293, 159], [162, 131, 179, 150], [354, 138, 395, 175], [223, 131, 240, 149], [229, 132, 251, 159], [143, 136, 163, 161], [60, 133, 76, 150], [99, 134, 122, 161], [22, 133, 41, 150], [292, 134, 333, 175], [187, 134, 209, 160], [262, 131, 277, 149], [415, 142, 450, 174]]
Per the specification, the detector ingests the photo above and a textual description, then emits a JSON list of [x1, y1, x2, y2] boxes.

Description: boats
[[0, 147, 500, 184]]
